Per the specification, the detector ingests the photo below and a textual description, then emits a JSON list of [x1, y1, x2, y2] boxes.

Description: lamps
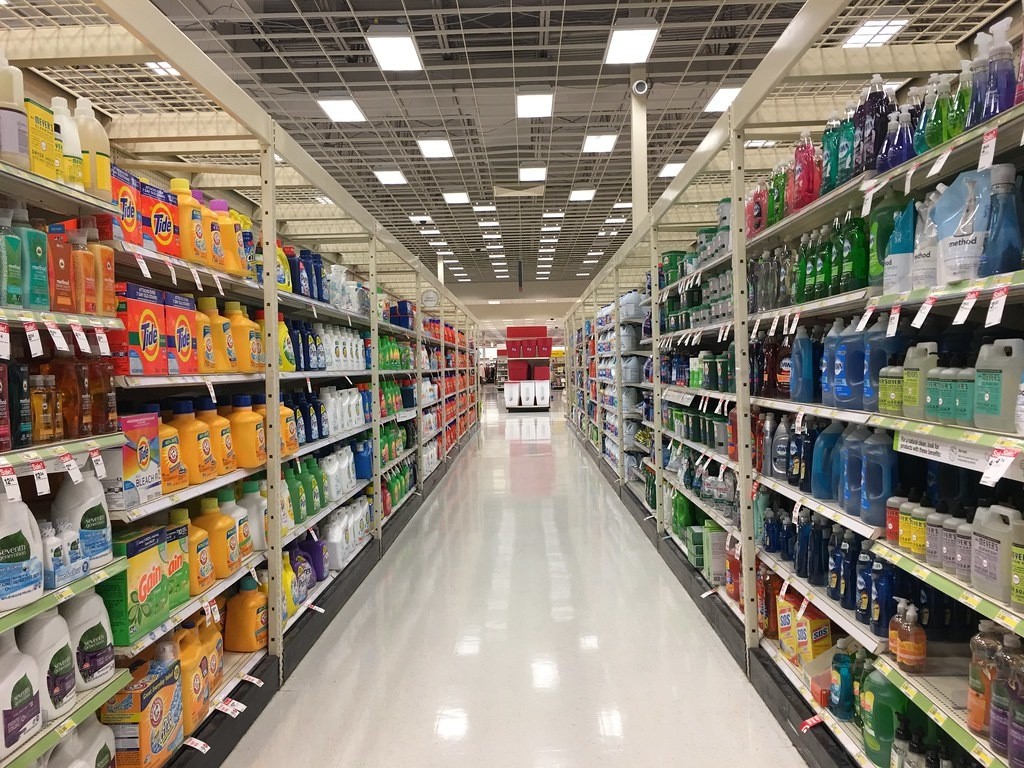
[[515, 83, 554, 119], [415, 131, 455, 159], [362, 22, 425, 75], [602, 16, 663, 66], [568, 182, 596, 203], [519, 160, 547, 183], [580, 126, 620, 153], [370, 164, 408, 186], [313, 89, 368, 125]]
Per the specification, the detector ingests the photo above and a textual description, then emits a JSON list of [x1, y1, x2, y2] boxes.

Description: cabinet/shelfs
[[504, 326, 551, 413], [98, 238, 482, 768], [495, 360, 509, 390], [637, 102, 1024, 768], [561, 214, 717, 549], [0, 160, 134, 768], [551, 357, 566, 389]]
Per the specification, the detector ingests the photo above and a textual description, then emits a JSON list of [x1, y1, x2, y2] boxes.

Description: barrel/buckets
[[748, 311, 911, 417], [763, 409, 896, 528], [975, 334, 1023, 434], [970, 497, 1022, 604]]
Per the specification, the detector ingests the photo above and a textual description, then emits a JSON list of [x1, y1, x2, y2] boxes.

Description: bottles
[[746, 197, 870, 312], [885, 481, 974, 582], [752, 481, 957, 639], [828, 635, 876, 736], [967, 618, 1024, 768]]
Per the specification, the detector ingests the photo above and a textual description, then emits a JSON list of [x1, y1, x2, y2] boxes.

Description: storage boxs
[[166, 523, 190, 610], [704, 519, 718, 528], [792, 605, 832, 670], [686, 526, 703, 545], [707, 530, 737, 585], [96, 162, 143, 247], [95, 658, 183, 768], [107, 282, 163, 376], [775, 594, 803, 660], [95, 524, 171, 646], [24, 98, 57, 182], [687, 539, 703, 557], [701, 525, 724, 580], [140, 182, 182, 258], [811, 675, 831, 708], [802, 636, 863, 692], [163, 291, 198, 375], [97, 412, 162, 512], [688, 550, 704, 568]]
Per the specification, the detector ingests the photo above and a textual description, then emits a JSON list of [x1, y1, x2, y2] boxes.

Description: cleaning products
[[567, 16, 1024, 767], [0, 59, 477, 767]]
